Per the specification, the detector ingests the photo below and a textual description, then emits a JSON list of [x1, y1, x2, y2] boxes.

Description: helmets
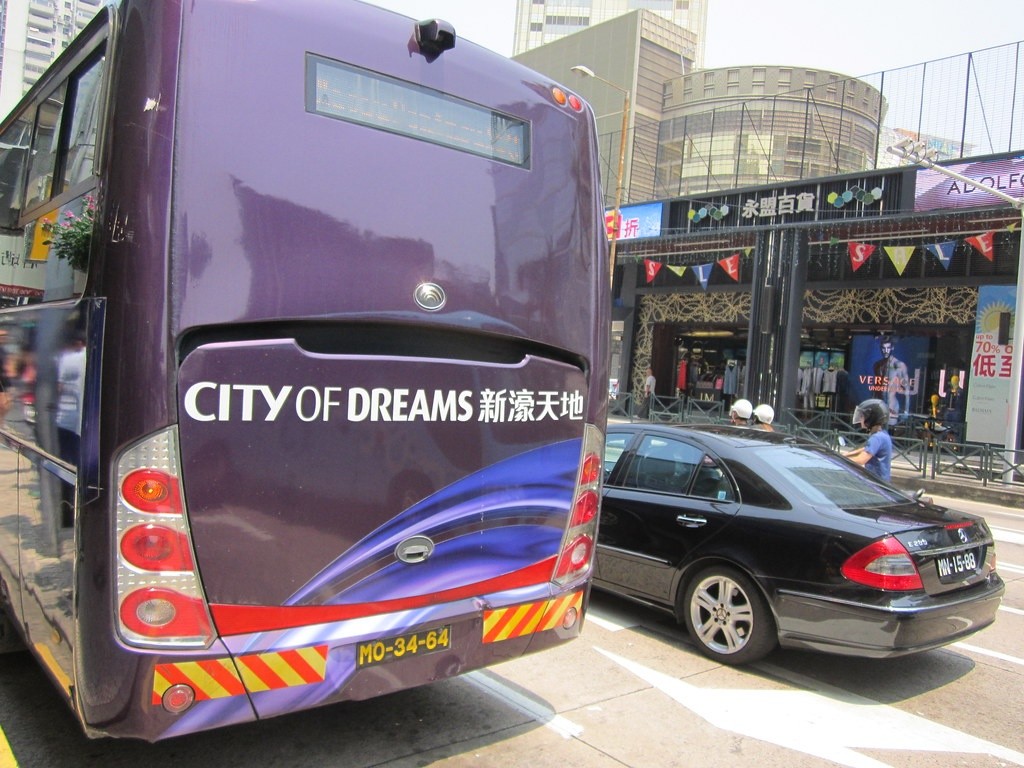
[[852, 398, 888, 432], [729, 399, 753, 420], [753, 403, 775, 424]]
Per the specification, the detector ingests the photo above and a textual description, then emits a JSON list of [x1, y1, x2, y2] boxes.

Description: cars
[[592, 423, 1003, 666]]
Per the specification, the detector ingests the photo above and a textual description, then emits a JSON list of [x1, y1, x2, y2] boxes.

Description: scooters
[[827, 435, 934, 506]]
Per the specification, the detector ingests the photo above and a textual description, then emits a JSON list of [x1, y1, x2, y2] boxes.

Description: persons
[[0, 326, 43, 471], [703, 398, 753, 478], [751, 403, 775, 433], [603, 433, 652, 488], [840, 398, 893, 485], [943, 375, 964, 451], [817, 355, 827, 371], [632, 368, 656, 420], [923, 394, 947, 447], [871, 337, 910, 426], [56, 328, 87, 528]]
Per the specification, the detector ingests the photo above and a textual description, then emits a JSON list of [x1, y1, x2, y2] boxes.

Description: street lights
[[570, 64, 631, 287]]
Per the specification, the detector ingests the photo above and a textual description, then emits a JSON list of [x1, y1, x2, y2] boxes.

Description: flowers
[[42, 194, 96, 270]]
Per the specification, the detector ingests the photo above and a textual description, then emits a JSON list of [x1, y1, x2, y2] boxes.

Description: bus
[[0, 0, 609, 745]]
